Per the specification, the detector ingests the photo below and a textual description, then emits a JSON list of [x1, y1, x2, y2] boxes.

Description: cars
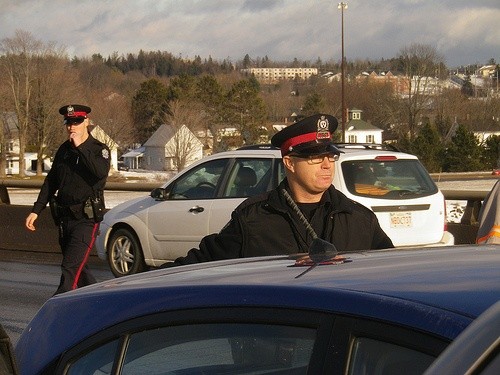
[[13, 243, 499, 375]]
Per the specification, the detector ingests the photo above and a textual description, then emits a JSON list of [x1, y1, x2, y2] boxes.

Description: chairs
[[230, 167, 257, 196]]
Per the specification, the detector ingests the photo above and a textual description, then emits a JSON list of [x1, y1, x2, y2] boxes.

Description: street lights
[[337, 2, 348, 149]]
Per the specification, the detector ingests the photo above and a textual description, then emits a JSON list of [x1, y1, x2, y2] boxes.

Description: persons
[[26, 104, 111, 297], [136, 114, 395, 273]]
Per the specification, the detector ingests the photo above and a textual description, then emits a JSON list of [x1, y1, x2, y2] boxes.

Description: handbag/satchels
[[48, 196, 72, 226]]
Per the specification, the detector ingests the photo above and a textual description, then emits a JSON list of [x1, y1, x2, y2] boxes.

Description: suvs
[[95, 143, 455, 278]]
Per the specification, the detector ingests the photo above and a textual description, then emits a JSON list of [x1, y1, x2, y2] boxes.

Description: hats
[[59, 104, 91, 125], [271, 114, 345, 156]]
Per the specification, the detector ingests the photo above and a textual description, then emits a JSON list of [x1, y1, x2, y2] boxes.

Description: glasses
[[288, 153, 340, 163]]
